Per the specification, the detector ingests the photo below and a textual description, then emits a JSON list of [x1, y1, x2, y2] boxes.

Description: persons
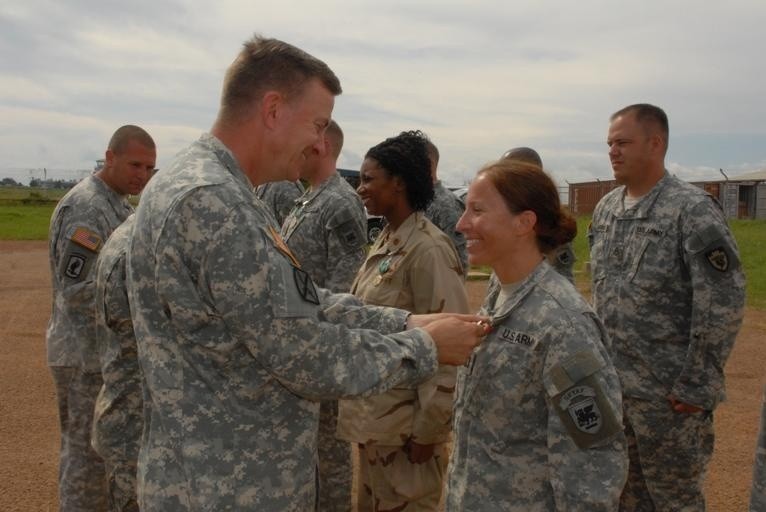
[[280, 119, 370, 511], [587, 103, 749, 512], [441, 158, 630, 510], [420, 138, 468, 288], [43, 126, 155, 511], [87, 212, 144, 511], [125, 35, 493, 510], [499, 147, 576, 291], [252, 180, 305, 230], [749, 403, 766, 511]]
